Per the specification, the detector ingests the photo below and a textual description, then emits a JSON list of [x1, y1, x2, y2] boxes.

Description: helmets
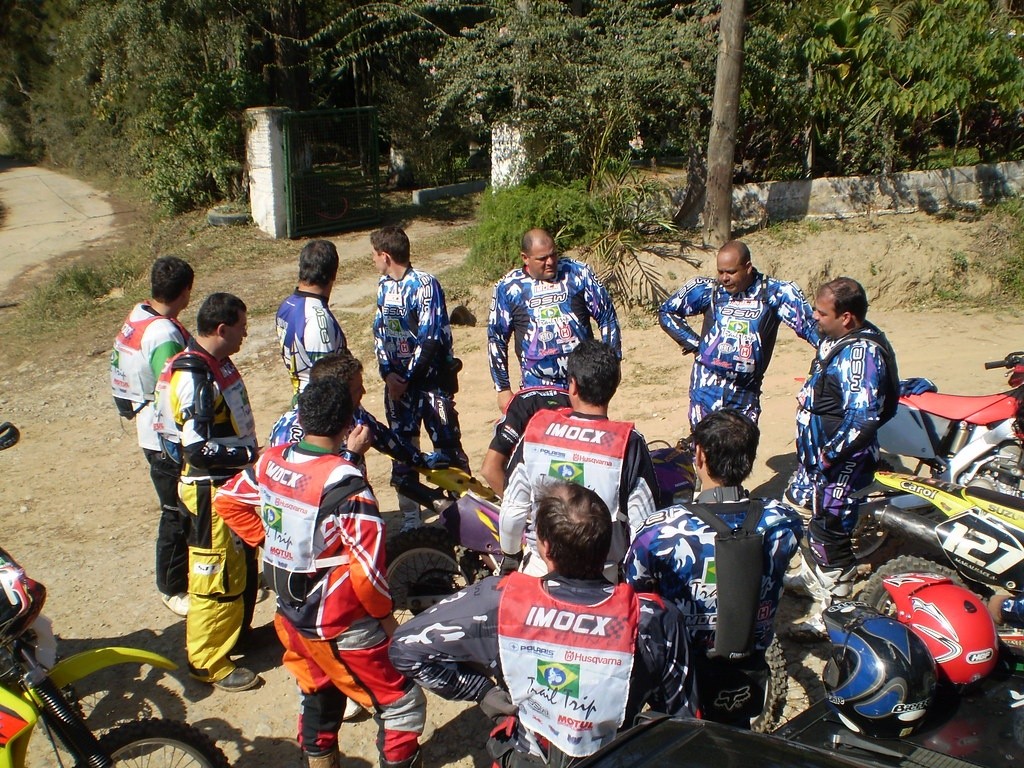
[[881, 572, 997, 691], [822, 603, 937, 739]]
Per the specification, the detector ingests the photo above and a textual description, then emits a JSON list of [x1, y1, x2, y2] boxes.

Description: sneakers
[[208, 666, 259, 691], [230, 625, 278, 656], [161, 591, 190, 615]]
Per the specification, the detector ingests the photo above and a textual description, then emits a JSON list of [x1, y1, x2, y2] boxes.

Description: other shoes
[[255, 588, 266, 604], [342, 697, 363, 720]]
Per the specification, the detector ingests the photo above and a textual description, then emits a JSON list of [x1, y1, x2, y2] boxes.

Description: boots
[[301, 741, 342, 768], [379, 742, 424, 768], [787, 565, 857, 640], [398, 494, 422, 533], [782, 553, 822, 602]]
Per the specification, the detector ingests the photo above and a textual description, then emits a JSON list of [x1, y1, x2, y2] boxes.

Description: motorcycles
[[363, 349, 1023, 767], [1, 421, 237, 768]]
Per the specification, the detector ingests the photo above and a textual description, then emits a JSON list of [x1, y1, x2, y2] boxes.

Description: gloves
[[499, 550, 524, 575], [480, 687, 520, 738], [899, 377, 938, 397]]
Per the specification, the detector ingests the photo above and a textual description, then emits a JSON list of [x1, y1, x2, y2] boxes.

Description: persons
[[495, 338, 659, 586], [277, 235, 348, 408], [153, 293, 258, 692], [369, 224, 473, 490], [113, 255, 198, 616], [487, 230, 622, 414], [482, 384, 575, 497], [266, 353, 453, 719], [210, 373, 426, 768], [781, 279, 899, 647], [389, 478, 700, 767], [655, 241, 825, 492], [622, 409, 803, 728]]
